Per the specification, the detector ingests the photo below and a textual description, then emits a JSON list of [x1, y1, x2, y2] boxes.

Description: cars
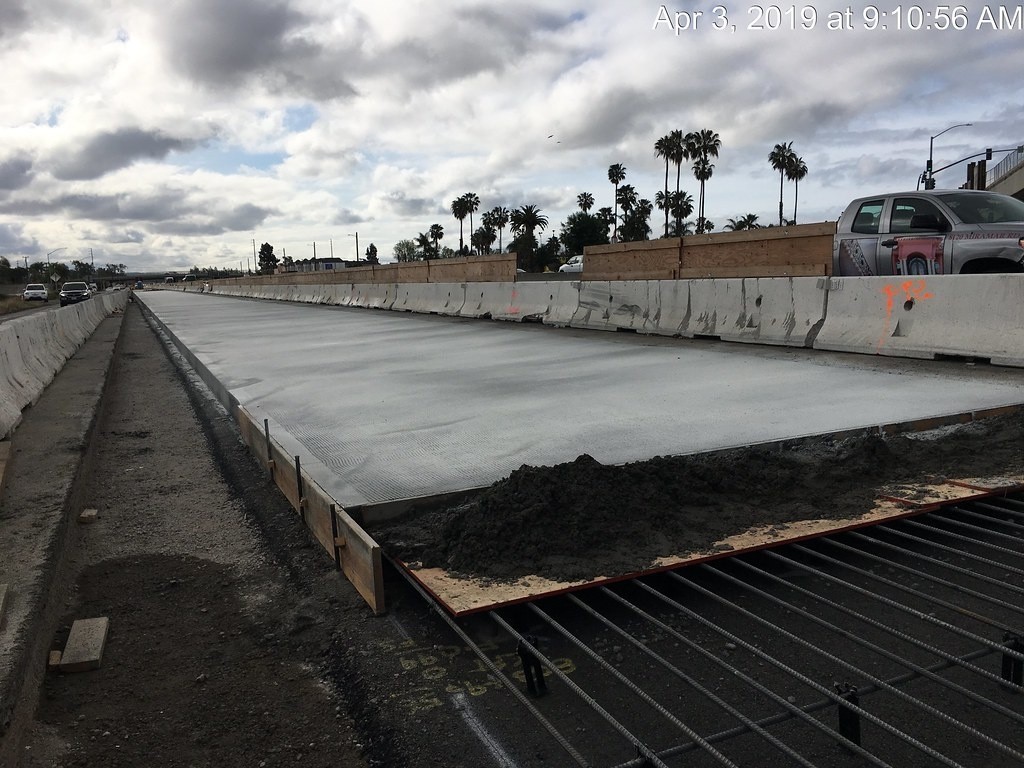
[[87, 282, 97, 295], [166, 277, 174, 283], [557, 255, 584, 273], [106, 280, 143, 293], [22, 284, 49, 303], [182, 274, 196, 282], [58, 281, 92, 307], [830, 187, 1024, 277]]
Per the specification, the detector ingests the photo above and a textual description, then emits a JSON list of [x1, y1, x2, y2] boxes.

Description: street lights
[[47, 247, 68, 284], [305, 241, 317, 271], [347, 231, 359, 267], [930, 124, 973, 159]]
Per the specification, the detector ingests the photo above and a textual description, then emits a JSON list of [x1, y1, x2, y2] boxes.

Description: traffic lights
[[930, 178, 936, 189], [927, 160, 933, 172]]
